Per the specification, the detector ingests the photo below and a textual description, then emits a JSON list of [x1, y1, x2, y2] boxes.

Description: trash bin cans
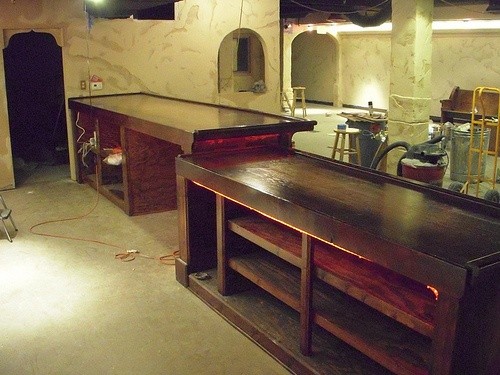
[[449, 125, 490, 183]]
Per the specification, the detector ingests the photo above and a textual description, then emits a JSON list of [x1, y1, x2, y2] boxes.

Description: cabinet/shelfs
[[174, 142, 500, 375]]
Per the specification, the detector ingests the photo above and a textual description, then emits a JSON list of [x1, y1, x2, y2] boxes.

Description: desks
[[68, 92, 317, 216], [337, 112, 387, 167]]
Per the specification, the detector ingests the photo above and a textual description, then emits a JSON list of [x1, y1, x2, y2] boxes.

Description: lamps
[[283, 17, 292, 30]]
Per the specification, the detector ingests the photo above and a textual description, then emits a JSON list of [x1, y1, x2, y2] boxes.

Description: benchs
[[440, 86, 499, 124]]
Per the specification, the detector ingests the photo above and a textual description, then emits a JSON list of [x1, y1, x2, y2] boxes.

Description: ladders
[[0, 195, 18, 242], [282, 91, 292, 113]]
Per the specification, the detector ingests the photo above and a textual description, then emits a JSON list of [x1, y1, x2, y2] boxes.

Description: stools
[[291, 87, 307, 118], [332, 128, 361, 164]]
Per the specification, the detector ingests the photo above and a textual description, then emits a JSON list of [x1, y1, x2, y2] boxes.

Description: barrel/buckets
[[449, 126, 491, 183]]
